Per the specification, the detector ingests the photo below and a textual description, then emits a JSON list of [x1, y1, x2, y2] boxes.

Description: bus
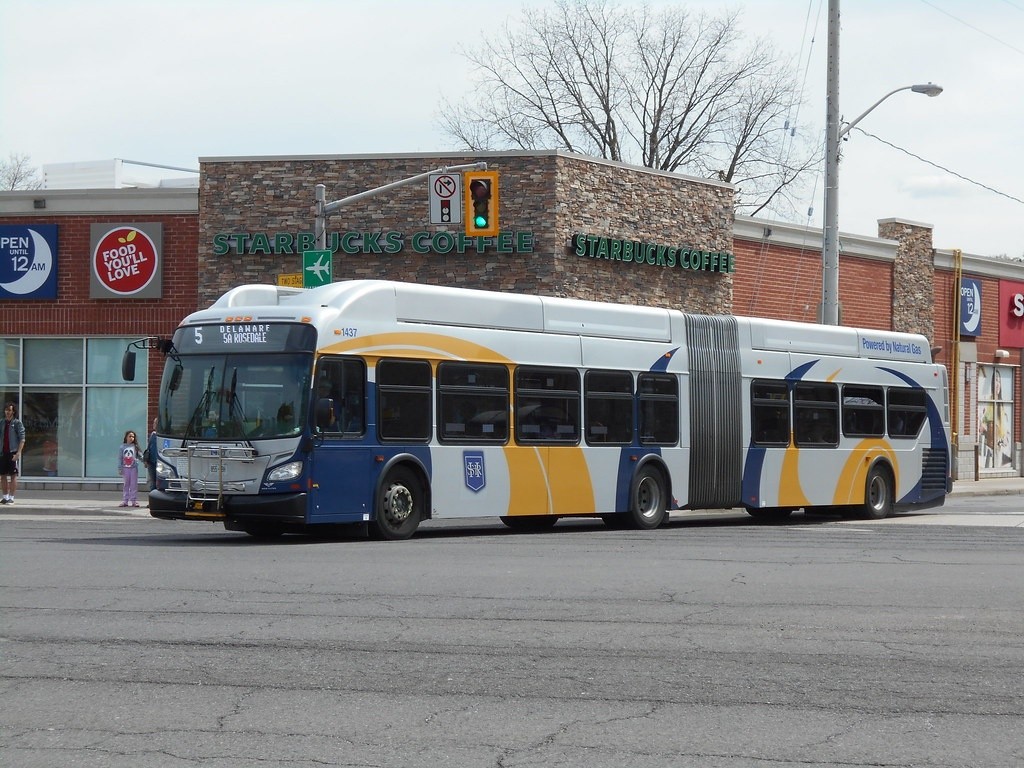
[[119, 276, 956, 540]]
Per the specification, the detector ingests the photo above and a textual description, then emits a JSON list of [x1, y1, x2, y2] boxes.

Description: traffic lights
[[464, 168, 499, 239]]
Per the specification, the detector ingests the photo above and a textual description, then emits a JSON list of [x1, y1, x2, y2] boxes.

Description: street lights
[[820, 1, 943, 328]]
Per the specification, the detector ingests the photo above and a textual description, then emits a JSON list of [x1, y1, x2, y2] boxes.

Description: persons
[[316, 381, 340, 432], [203, 408, 224, 438], [979, 369, 1010, 468], [118, 416, 159, 508], [0, 402, 25, 506], [277, 400, 291, 433]]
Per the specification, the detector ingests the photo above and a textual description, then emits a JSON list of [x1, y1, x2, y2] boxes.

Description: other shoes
[[119, 502, 128, 507], [132, 502, 140, 507], [4, 499, 14, 504], [0, 498, 9, 505]]
